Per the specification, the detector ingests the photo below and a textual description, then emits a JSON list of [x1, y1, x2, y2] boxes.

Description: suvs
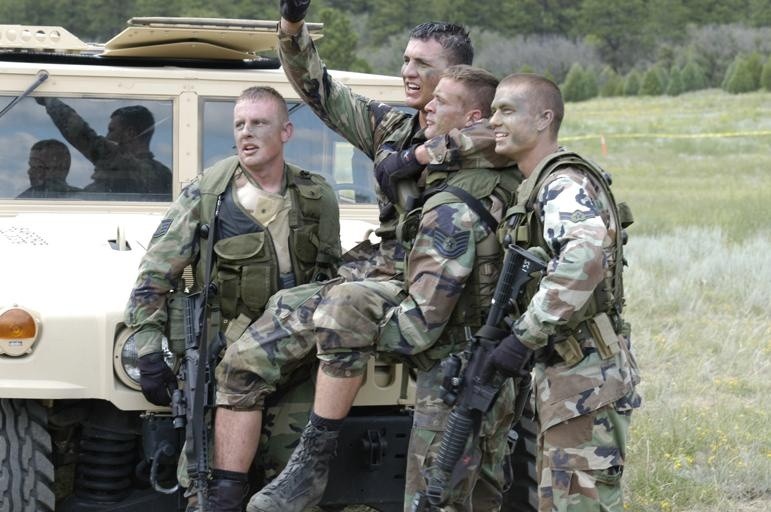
[[0, 16, 539, 512]]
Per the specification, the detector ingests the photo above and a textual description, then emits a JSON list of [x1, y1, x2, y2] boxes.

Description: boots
[[187, 466, 252, 510], [245, 420, 340, 511]]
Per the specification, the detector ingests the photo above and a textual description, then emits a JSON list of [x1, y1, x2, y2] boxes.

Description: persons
[[120, 86, 346, 509], [482, 71, 647, 510], [13, 134, 85, 198], [32, 96, 176, 195], [200, 0, 520, 510], [359, 62, 536, 510]]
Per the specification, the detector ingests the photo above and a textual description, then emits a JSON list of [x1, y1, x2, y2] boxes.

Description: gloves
[[135, 350, 179, 408], [277, 0, 312, 25], [374, 140, 429, 207], [479, 328, 531, 389]]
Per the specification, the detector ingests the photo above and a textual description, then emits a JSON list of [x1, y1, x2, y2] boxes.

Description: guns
[[412, 243, 546, 512], [169, 285, 225, 512]]
